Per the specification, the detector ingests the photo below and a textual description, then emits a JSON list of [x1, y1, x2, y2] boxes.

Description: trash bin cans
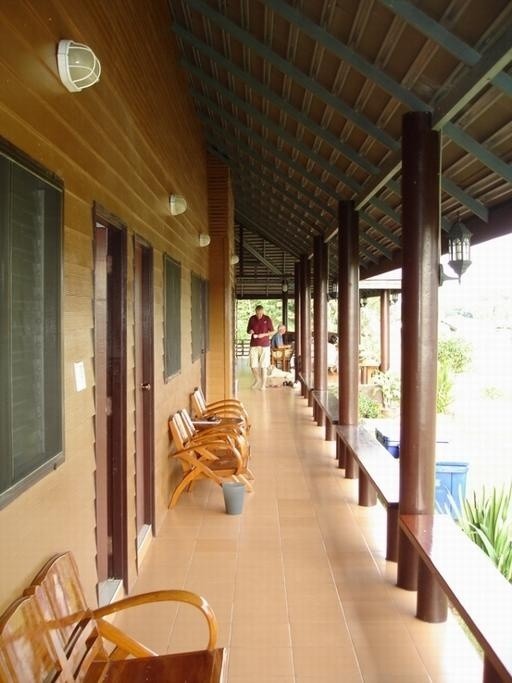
[[374, 426, 468, 521], [223, 482, 245, 516]]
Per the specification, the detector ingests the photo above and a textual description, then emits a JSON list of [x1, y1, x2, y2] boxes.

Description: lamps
[[169, 191, 241, 265], [446, 213, 474, 281], [58, 35, 102, 93]]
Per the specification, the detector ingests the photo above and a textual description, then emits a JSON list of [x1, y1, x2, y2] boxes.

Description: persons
[[271, 323, 295, 368], [246, 303, 275, 392]]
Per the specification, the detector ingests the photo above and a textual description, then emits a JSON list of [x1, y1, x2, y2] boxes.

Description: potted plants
[[370, 368, 401, 417]]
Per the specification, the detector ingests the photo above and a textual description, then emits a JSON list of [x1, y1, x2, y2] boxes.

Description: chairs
[[167, 384, 253, 512], [272, 345, 291, 370], [2, 549, 226, 681]]
